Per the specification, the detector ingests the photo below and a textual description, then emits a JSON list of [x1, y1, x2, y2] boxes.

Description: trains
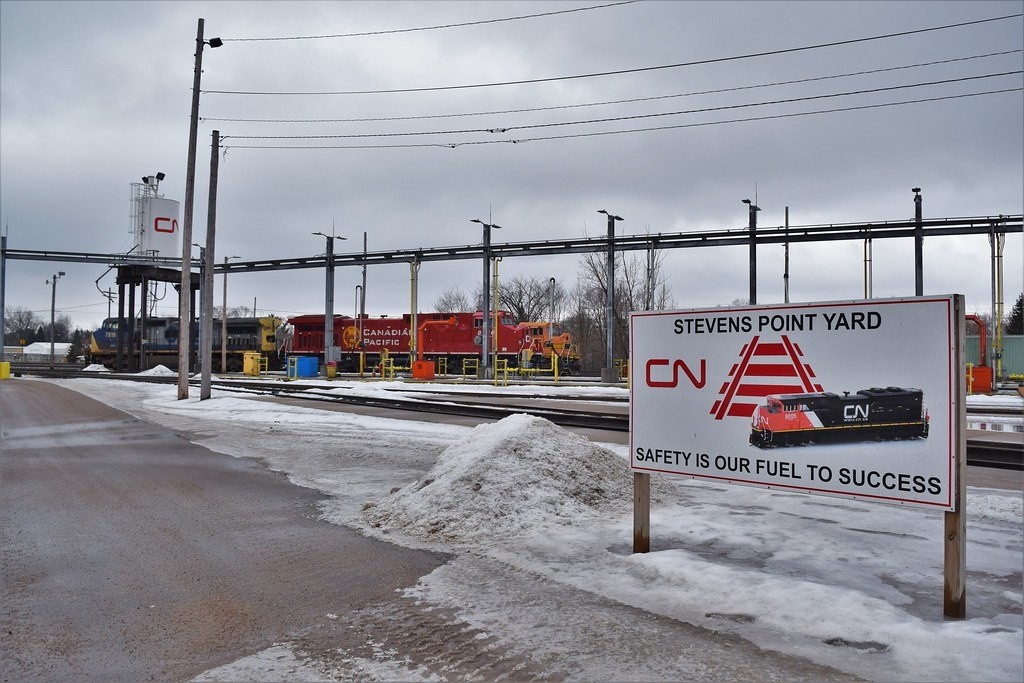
[[91, 310, 539, 371], [749, 388, 929, 450], [518, 321, 582, 376]]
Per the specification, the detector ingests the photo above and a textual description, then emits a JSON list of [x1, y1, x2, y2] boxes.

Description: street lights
[[45, 271, 65, 364], [470, 219, 502, 367], [597, 209, 625, 369], [742, 198, 761, 306], [311, 233, 345, 364], [911, 186, 924, 295]]
